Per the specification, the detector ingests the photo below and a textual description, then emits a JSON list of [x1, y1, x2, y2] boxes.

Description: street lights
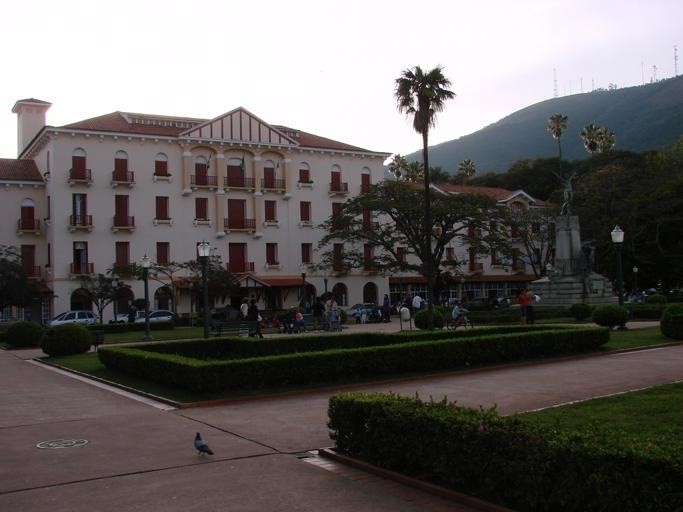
[[197, 238, 211, 338], [139, 250, 152, 338], [611, 224, 625, 306]]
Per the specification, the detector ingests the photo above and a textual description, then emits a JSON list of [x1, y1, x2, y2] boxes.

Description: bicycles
[[447, 311, 473, 330]]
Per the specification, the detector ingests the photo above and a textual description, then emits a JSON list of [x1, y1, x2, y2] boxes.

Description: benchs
[[216, 321, 286, 336]]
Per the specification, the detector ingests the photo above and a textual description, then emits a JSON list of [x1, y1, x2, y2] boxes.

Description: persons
[[518, 288, 529, 326], [452, 299, 471, 325], [238, 294, 391, 337], [527, 291, 536, 324], [411, 295, 425, 312], [121, 304, 137, 323]]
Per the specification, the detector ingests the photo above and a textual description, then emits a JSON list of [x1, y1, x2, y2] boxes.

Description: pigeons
[[193, 431, 215, 456]]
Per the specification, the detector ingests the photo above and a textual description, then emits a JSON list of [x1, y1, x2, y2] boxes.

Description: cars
[[345, 303, 379, 314], [110, 310, 174, 325], [46, 310, 98, 326]]
